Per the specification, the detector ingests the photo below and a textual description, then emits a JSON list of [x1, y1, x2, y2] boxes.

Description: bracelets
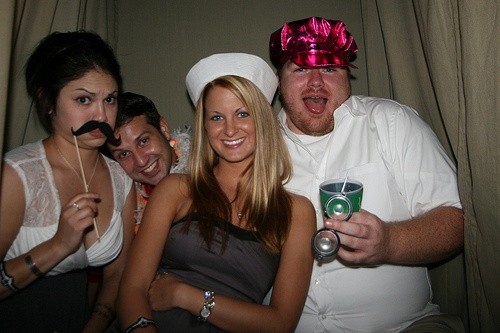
[[195, 289, 216, 323], [120, 317, 157, 333], [0, 262, 19, 294], [22, 252, 45, 279]]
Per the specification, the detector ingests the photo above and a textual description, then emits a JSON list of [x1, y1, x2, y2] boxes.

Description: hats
[[184, 52, 279, 106], [269, 16, 361, 71]]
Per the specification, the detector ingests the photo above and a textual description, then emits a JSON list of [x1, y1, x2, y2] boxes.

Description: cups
[[319, 179, 364, 233]]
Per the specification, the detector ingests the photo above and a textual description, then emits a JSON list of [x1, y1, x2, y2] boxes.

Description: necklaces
[[221, 184, 249, 220], [51, 137, 100, 189]]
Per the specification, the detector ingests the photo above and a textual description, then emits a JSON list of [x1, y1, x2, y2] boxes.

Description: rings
[[73, 203, 80, 211]]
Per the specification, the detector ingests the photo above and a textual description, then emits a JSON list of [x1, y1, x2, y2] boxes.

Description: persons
[[0, 31, 138, 333], [115, 53, 318, 333], [100, 91, 192, 237], [267, 16, 465, 333]]
